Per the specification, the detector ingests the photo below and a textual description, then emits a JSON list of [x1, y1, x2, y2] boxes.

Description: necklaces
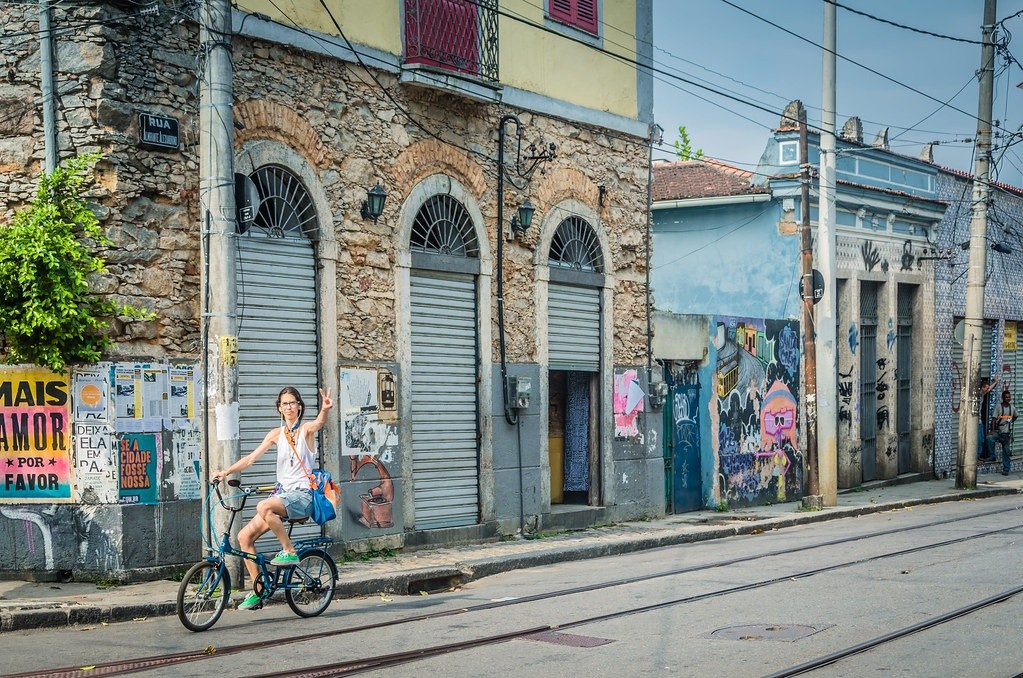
[[286, 421, 301, 467]]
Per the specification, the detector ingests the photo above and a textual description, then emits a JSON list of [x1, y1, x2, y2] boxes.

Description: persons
[[210, 385, 333, 611], [985, 391, 1018, 476], [977, 374, 998, 465]]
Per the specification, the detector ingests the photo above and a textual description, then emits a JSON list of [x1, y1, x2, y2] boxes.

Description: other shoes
[[985, 457, 996, 461], [977, 459, 983, 465], [1002, 470, 1008, 476]]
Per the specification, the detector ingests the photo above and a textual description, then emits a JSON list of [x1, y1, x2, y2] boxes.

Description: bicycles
[[177, 478, 341, 632]]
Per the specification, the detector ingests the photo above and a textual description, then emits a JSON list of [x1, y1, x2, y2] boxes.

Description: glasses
[[278, 400, 300, 406]]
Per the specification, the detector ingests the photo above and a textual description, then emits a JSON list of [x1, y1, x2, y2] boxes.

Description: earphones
[[299, 405, 302, 411], [279, 407, 282, 412]]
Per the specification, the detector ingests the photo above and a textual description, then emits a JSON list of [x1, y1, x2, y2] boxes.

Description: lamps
[[511, 196, 536, 238], [359, 179, 389, 225]]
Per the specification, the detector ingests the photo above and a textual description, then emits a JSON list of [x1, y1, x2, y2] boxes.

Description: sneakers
[[270, 549, 300, 566], [238, 590, 271, 610]]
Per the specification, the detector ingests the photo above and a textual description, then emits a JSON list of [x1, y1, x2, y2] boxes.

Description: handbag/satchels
[[999, 432, 1010, 445], [309, 469, 340, 525]]
